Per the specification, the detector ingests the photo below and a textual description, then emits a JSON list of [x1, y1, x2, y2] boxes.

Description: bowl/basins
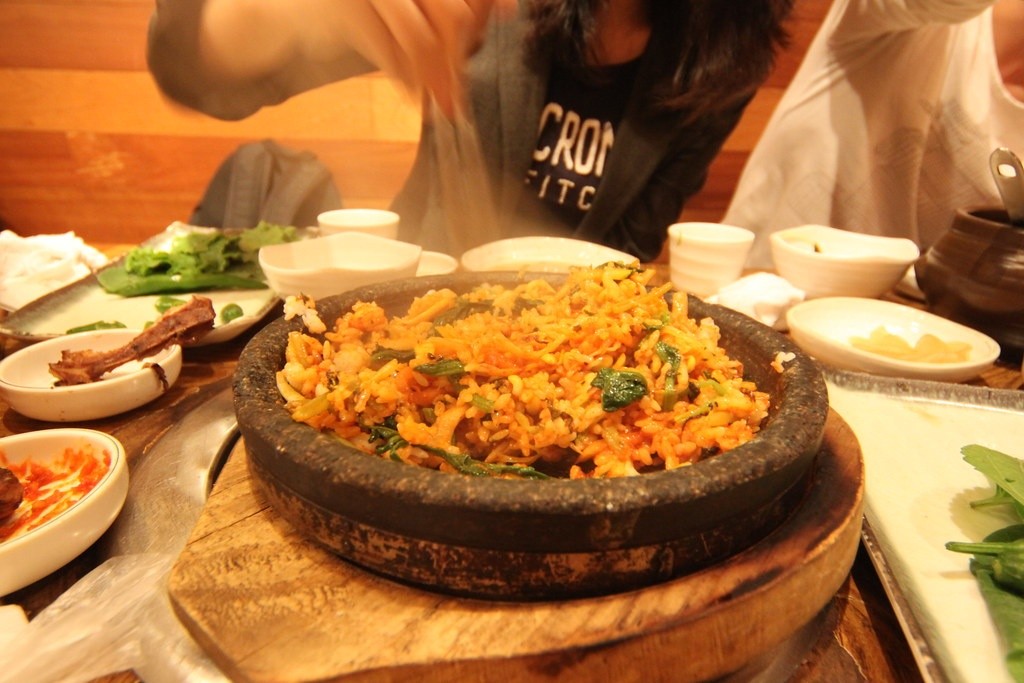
[[914, 203, 1024, 363], [769, 224, 919, 301], [232, 271, 827, 600], [260, 231, 422, 301]]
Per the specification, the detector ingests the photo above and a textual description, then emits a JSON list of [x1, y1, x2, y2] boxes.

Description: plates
[[787, 296, 1000, 382], [0, 428, 129, 596], [1, 221, 281, 346], [461, 235, 640, 273], [810, 357, 1024, 683], [417, 250, 457, 278], [0, 328, 182, 422]]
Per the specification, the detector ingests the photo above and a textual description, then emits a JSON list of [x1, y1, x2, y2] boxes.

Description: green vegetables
[[943, 442, 1024, 683], [354, 294, 702, 482], [65, 221, 313, 335]]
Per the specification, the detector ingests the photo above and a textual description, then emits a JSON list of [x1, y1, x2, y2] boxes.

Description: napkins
[[0, 230, 109, 313]]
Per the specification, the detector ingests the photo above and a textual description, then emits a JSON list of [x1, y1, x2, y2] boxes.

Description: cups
[[668, 222, 755, 298], [316, 209, 400, 241]]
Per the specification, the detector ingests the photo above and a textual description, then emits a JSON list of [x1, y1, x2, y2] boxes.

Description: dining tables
[[0, 242, 1024, 683]]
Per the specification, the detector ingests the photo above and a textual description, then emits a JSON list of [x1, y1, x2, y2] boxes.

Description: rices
[[271, 263, 773, 481]]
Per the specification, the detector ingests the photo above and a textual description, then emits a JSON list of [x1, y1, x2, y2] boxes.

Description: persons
[[142, 0, 1023, 264]]
